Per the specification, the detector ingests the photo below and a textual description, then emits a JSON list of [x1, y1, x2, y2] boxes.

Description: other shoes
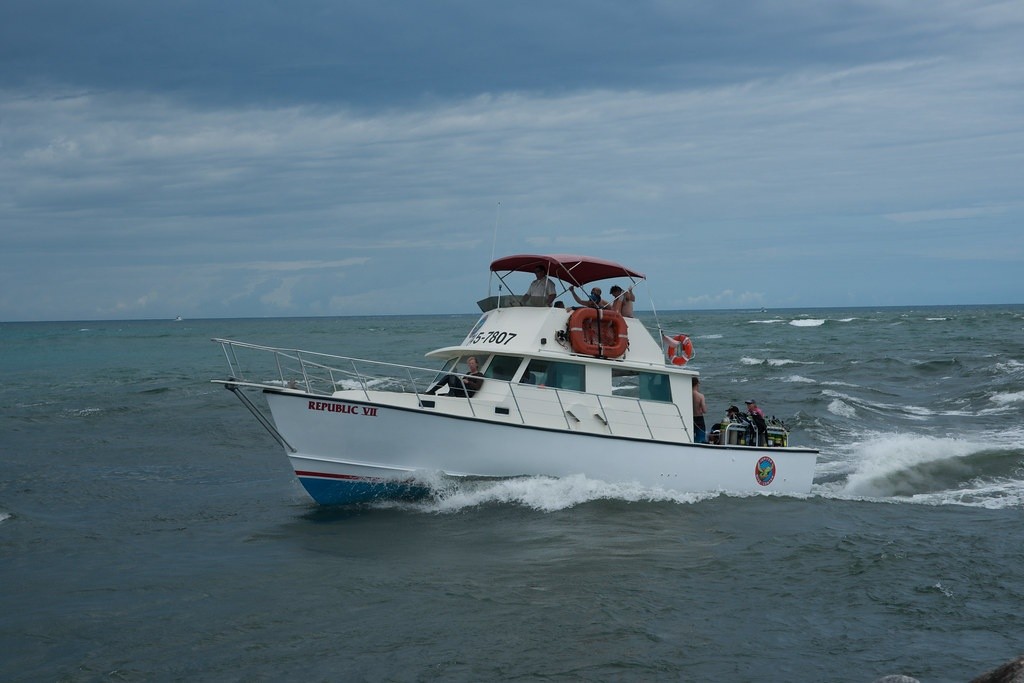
[[424, 390, 436, 395]]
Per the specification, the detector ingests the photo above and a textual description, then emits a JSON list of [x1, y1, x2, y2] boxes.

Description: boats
[[211, 254, 820, 511]]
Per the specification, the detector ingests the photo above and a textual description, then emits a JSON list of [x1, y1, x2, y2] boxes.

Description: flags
[[661, 331, 680, 348]]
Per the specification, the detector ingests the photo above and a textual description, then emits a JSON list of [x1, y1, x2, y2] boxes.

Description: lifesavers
[[668, 335, 693, 365]]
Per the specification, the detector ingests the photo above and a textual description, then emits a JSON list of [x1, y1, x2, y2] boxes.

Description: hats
[[692, 377, 700, 384], [745, 399, 755, 403], [725, 405, 739, 412]]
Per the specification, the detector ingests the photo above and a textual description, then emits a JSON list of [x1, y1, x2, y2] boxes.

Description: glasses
[[747, 403, 752, 405], [535, 270, 542, 274]]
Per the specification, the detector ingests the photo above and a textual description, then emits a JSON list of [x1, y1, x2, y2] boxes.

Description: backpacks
[[750, 413, 767, 438]]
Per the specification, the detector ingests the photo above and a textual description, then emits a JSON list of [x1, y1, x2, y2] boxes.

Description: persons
[[692, 376, 708, 442], [725, 406, 739, 418], [519, 266, 556, 308], [554, 301, 572, 312], [424, 357, 485, 398], [647, 374, 671, 401], [519, 367, 536, 384], [569, 285, 612, 309], [609, 284, 635, 317], [745, 399, 765, 418]]
